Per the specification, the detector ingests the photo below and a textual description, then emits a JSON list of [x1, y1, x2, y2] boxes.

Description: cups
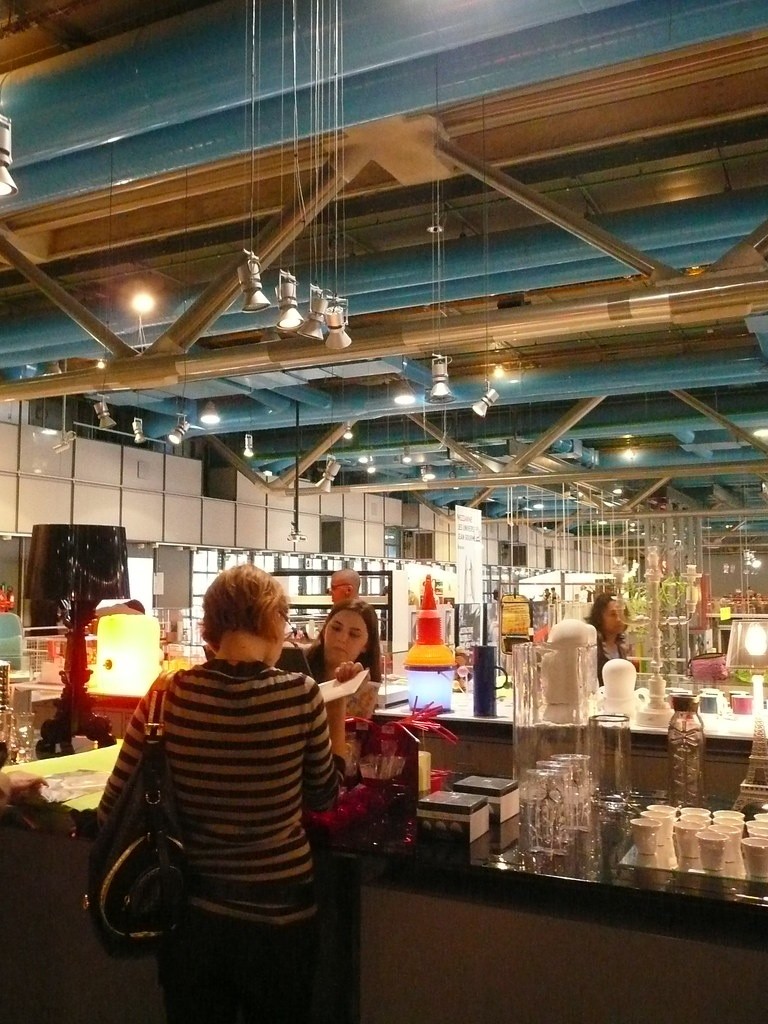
[[666, 687, 693, 698], [552, 754, 593, 833], [587, 714, 630, 810], [0, 711, 38, 763], [699, 687, 725, 713], [630, 804, 768, 877], [528, 770, 572, 855], [536, 760, 574, 828], [474, 646, 508, 717]]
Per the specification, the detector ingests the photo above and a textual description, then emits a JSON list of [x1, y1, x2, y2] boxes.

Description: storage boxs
[[416, 791, 492, 844], [452, 775, 522, 823]]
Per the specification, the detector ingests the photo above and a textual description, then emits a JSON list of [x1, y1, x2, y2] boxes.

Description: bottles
[[667, 695, 707, 815], [730, 691, 753, 714], [519, 770, 539, 856]]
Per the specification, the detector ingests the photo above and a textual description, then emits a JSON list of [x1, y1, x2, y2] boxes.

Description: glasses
[[331, 583, 354, 591]]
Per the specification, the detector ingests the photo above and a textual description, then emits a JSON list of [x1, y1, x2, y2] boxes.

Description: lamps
[[200, 401, 221, 424], [243, 396, 255, 457], [315, 459, 341, 494], [425, 53, 501, 419], [236, 0, 355, 353], [94, 124, 196, 450], [0, 0, 23, 195], [742, 474, 762, 569]]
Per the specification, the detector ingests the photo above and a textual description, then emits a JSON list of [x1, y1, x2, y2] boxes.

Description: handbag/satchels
[[82, 824, 192, 948]]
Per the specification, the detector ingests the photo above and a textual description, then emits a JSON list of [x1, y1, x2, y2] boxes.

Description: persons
[[29, 599, 62, 637], [94, 599, 146, 617], [327, 567, 361, 606], [719, 586, 765, 612], [541, 584, 595, 605], [97, 563, 370, 1024], [588, 591, 631, 689], [303, 597, 384, 682]]
